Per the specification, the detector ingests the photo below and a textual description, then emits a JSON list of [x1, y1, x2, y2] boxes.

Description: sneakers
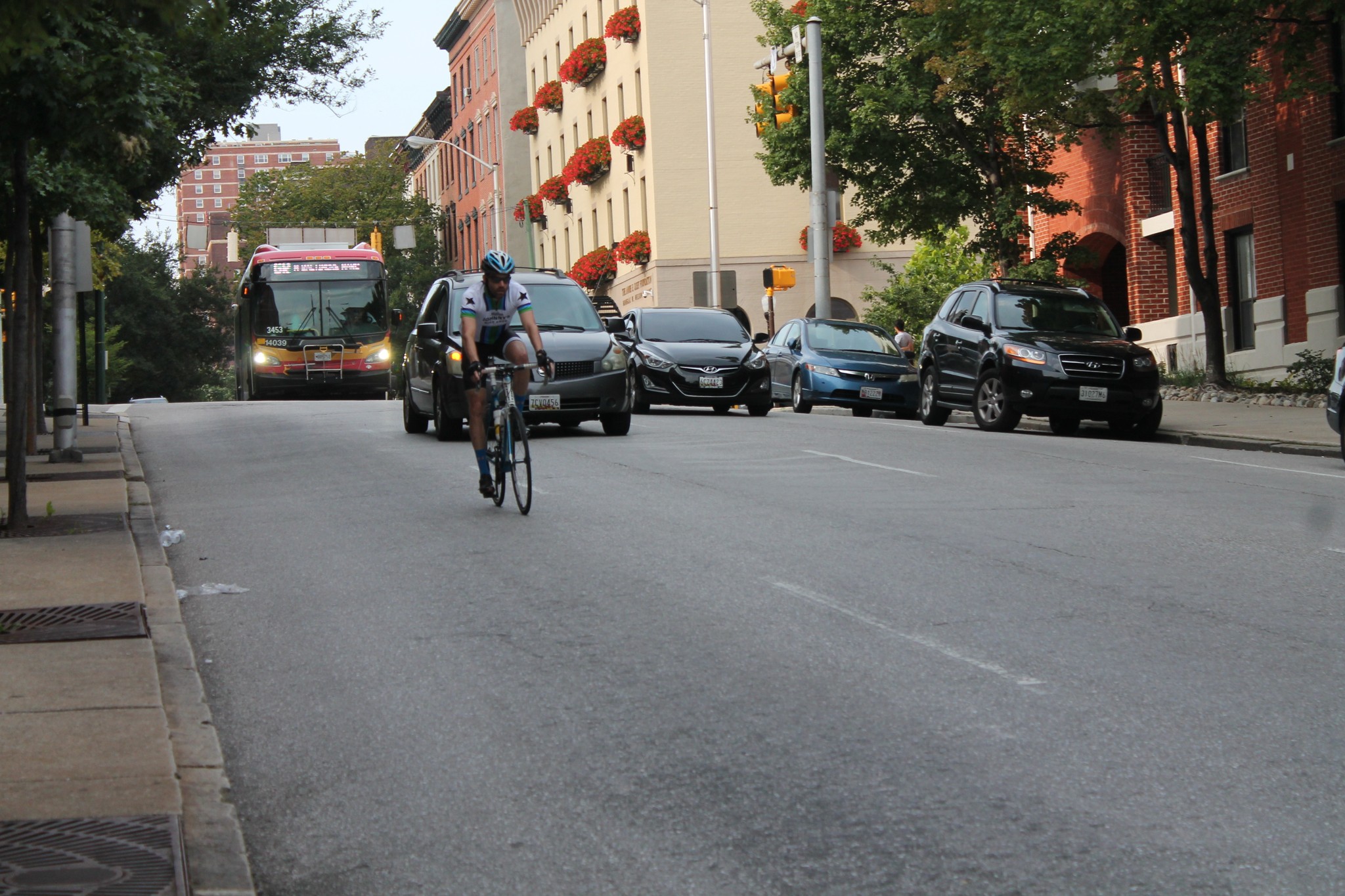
[[479, 474, 495, 495], [506, 460, 516, 472]]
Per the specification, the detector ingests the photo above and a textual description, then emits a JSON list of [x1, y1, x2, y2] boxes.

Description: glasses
[[488, 272, 512, 283]]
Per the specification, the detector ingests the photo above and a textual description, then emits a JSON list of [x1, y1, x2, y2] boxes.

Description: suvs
[[401, 266, 634, 443], [916, 276, 1164, 442]]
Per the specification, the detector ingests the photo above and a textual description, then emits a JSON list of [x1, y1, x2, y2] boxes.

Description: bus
[[225, 240, 404, 402]]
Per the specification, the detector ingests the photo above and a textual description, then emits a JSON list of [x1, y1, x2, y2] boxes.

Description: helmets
[[480, 249, 515, 274]]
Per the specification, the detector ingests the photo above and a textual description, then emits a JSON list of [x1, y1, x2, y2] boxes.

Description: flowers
[[509, 80, 563, 131], [604, 5, 640, 41], [799, 221, 862, 251], [561, 135, 612, 186], [513, 194, 543, 221], [564, 230, 652, 288], [537, 174, 570, 201], [557, 35, 606, 84], [610, 115, 646, 149]]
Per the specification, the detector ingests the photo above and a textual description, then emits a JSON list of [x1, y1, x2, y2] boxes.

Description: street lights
[[405, 136, 502, 254]]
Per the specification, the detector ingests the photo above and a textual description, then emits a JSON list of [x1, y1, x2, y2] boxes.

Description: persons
[[892, 318, 914, 353], [460, 249, 556, 498]]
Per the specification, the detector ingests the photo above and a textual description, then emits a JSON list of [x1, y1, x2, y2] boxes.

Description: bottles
[[500, 406, 507, 438], [493, 410, 504, 440]]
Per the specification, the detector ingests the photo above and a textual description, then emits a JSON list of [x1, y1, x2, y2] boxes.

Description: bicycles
[[460, 349, 553, 516]]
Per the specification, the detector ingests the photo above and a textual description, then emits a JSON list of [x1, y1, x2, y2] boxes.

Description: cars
[[757, 316, 921, 420], [608, 305, 773, 417]]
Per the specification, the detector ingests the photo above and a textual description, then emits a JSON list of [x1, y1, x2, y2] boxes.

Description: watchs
[[536, 349, 547, 356]]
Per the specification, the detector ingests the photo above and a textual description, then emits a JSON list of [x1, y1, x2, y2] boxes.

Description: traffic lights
[[772, 70, 799, 133], [752, 81, 776, 138], [762, 265, 796, 289]]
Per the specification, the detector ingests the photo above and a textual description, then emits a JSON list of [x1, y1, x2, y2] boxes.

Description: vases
[[587, 271, 615, 290], [833, 244, 848, 252], [549, 102, 561, 113], [630, 144, 642, 150], [578, 61, 605, 87], [555, 195, 567, 204], [623, 31, 637, 43], [526, 126, 537, 135], [582, 161, 609, 184], [633, 253, 648, 265]]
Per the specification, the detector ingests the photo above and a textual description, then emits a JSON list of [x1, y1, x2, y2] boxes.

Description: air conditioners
[[464, 87, 472, 97]]
